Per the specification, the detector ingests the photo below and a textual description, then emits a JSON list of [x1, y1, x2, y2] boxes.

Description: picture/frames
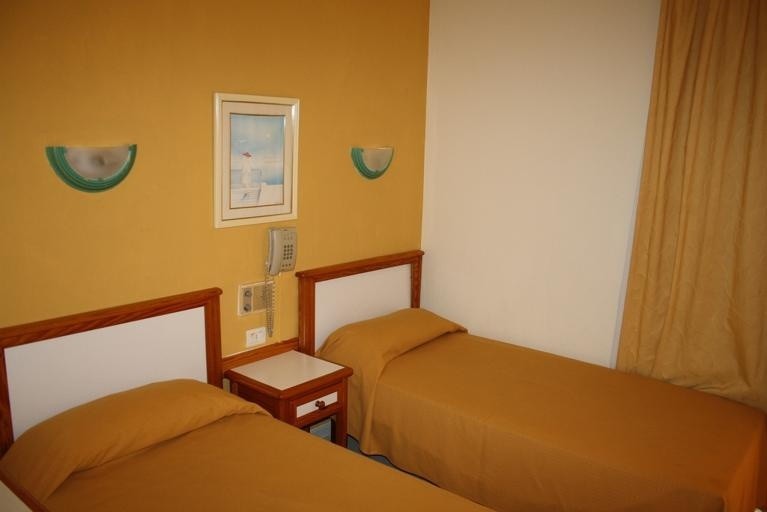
[[213, 91, 301, 227]]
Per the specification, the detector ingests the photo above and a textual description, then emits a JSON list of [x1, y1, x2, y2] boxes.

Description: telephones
[[267, 227, 297, 276]]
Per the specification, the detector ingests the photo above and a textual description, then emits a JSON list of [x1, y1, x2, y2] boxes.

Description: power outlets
[[246, 327, 266, 347]]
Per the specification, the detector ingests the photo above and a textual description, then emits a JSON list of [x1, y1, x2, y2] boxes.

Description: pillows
[[318, 307, 467, 383], [0, 379, 272, 504]]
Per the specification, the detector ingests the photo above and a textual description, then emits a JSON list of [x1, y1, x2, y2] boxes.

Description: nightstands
[[225, 348, 353, 448]]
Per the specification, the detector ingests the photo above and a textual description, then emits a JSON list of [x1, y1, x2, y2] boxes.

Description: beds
[[296, 250, 767, 512], [0, 287, 499, 512]]
